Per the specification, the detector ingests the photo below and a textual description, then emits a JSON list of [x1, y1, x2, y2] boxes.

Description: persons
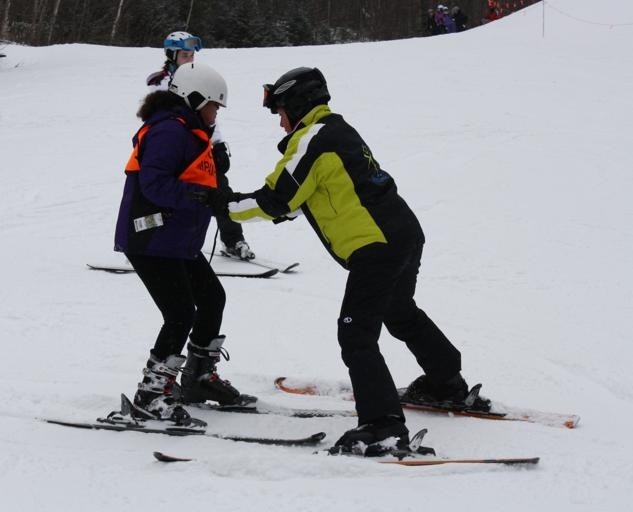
[[146, 29, 254, 263], [114, 62, 253, 427], [220, 66, 468, 451]]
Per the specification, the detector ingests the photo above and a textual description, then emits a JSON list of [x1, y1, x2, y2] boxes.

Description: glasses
[[165, 37, 201, 52]]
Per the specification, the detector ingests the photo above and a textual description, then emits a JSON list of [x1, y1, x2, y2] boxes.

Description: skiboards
[[34, 397, 359, 445], [86, 250, 299, 278], [154, 379, 580, 466]]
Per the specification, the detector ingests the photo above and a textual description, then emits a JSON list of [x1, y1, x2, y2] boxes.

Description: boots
[[181, 334, 240, 405], [134, 349, 191, 426]]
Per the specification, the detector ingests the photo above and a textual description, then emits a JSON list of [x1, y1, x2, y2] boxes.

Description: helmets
[[262, 67, 330, 129], [164, 32, 201, 62], [169, 63, 227, 111]]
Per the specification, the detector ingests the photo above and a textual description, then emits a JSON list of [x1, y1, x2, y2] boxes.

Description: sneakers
[[335, 422, 410, 450], [402, 375, 469, 405], [226, 239, 255, 260]]
